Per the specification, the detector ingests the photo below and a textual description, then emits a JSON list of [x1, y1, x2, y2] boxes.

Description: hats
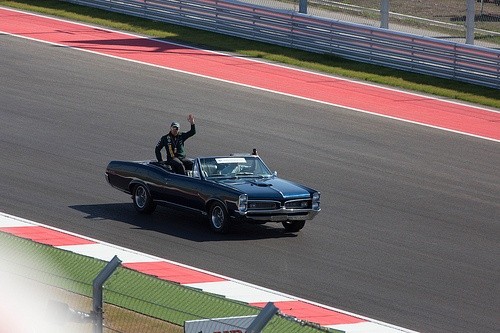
[[171, 122, 179, 128]]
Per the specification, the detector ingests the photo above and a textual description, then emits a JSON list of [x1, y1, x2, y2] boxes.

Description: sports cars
[[104, 152, 321, 234]]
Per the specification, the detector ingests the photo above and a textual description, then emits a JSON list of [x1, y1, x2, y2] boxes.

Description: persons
[[155, 114, 196, 175], [221, 151, 258, 174]]
